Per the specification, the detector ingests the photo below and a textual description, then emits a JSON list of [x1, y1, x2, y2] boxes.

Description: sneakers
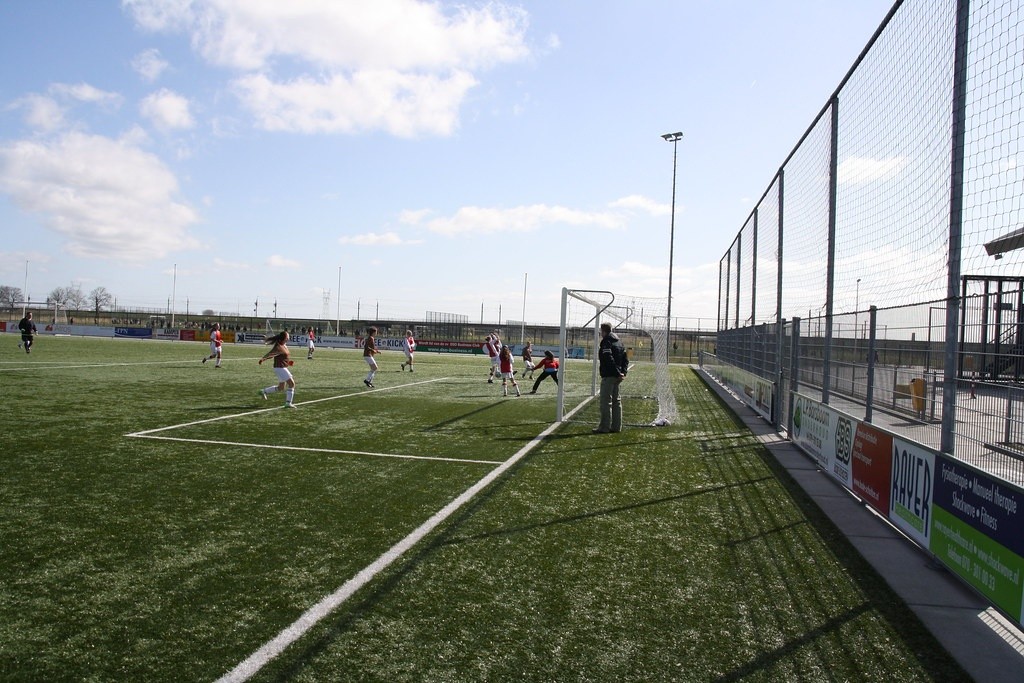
[[258, 389, 267, 401], [285, 401, 297, 409]]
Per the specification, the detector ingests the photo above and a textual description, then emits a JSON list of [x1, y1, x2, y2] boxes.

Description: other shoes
[[202, 358, 206, 363], [488, 379, 493, 383], [215, 365, 221, 368], [517, 392, 521, 396], [592, 428, 605, 433], [401, 364, 404, 371], [364, 380, 374, 388], [503, 392, 507, 396], [513, 370, 518, 375], [609, 429, 621, 433], [529, 375, 533, 380], [530, 390, 536, 394], [522, 374, 525, 379], [410, 369, 414, 372]]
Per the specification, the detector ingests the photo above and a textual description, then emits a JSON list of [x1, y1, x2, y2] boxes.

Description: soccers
[[496, 371, 502, 378]]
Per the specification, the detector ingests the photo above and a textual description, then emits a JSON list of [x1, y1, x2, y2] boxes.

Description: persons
[[334, 327, 361, 338], [178, 320, 248, 331], [364, 327, 382, 388], [17, 312, 38, 353], [592, 322, 629, 432], [202, 323, 223, 368], [522, 342, 535, 380], [485, 333, 521, 396], [259, 331, 297, 408], [530, 350, 559, 394], [308, 326, 315, 359], [112, 318, 172, 333], [285, 325, 322, 335], [402, 330, 415, 372]]
